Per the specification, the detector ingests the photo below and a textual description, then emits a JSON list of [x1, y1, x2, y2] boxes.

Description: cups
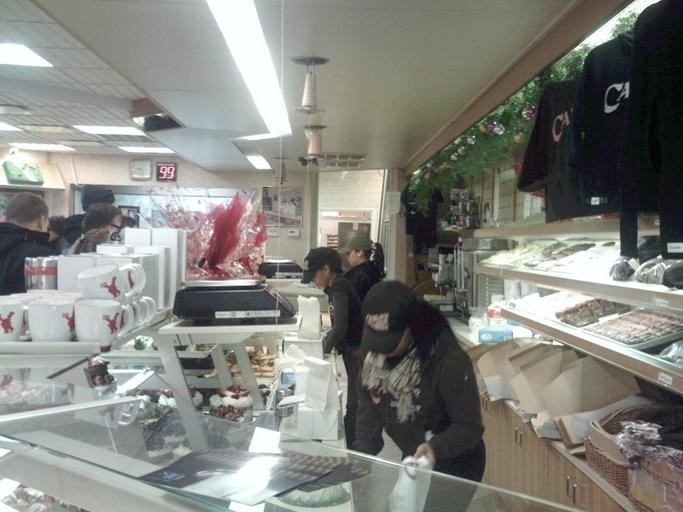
[[23, 257, 58, 291], [0, 263, 158, 344]]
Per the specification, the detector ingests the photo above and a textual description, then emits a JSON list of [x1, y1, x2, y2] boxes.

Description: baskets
[[581, 434, 632, 495]]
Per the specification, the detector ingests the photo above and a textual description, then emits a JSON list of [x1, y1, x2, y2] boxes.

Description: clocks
[[129, 158, 152, 180]]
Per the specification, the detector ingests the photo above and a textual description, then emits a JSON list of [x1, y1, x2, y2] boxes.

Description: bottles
[[468, 292, 534, 346], [449, 186, 482, 230]]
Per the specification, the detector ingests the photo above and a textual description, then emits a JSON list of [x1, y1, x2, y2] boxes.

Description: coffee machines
[[423, 243, 463, 317]]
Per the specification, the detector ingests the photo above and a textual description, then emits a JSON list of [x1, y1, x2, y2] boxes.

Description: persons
[[300, 248, 364, 472], [337, 235, 384, 302], [0, 185, 137, 295], [354, 282, 485, 512]]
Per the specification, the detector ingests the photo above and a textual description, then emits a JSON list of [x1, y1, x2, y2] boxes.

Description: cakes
[[119, 383, 253, 464]]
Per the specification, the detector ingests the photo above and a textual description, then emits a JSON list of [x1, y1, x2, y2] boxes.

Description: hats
[[336, 236, 373, 253], [79, 185, 116, 211], [301, 247, 338, 284], [360, 280, 413, 355]]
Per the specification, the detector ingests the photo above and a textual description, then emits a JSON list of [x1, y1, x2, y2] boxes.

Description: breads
[[555, 298, 683, 345], [227, 345, 277, 377]]
